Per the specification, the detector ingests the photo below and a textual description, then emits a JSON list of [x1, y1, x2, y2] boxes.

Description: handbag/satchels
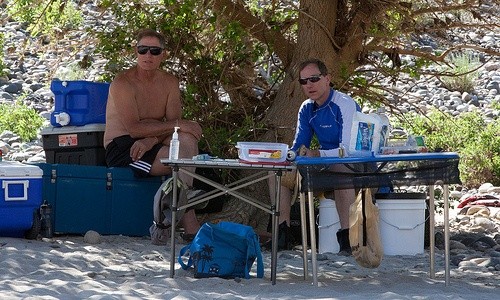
[[178, 221, 264, 279], [349, 188, 382, 269], [349, 111, 389, 156]]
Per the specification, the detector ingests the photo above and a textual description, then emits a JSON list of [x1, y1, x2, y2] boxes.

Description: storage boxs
[[236, 142, 288, 162], [0, 124, 169, 237]]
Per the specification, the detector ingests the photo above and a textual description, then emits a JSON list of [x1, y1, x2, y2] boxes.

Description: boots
[[260, 220, 294, 252], [336, 228, 352, 256]]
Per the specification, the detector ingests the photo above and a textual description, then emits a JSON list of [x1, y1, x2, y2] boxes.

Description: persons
[[264, 59, 361, 252], [104, 30, 202, 244]]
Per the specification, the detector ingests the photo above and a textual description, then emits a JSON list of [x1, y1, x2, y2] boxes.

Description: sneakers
[[185, 183, 209, 214], [179, 232, 196, 245]]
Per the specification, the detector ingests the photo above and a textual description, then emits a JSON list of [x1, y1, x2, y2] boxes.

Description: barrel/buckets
[[316, 199, 342, 254], [374, 192, 430, 256]]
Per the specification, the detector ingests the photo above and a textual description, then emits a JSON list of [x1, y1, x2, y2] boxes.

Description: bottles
[[40, 200, 54, 238], [376, 107, 390, 155]]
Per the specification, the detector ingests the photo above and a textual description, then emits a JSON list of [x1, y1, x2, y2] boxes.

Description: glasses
[[137, 45, 164, 56], [298, 73, 323, 85]]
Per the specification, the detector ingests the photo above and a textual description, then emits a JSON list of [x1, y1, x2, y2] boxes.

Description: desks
[[158, 157, 298, 285], [296, 151, 462, 287]]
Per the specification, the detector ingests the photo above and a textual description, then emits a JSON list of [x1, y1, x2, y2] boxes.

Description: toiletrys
[[168, 126, 180, 161]]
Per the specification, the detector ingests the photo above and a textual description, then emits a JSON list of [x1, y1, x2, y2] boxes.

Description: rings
[[139, 151, 141, 152]]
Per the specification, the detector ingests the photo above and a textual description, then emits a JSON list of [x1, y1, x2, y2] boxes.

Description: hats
[[277, 169, 298, 206]]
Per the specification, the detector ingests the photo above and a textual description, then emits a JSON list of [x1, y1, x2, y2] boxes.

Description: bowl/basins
[[235, 142, 289, 163]]
